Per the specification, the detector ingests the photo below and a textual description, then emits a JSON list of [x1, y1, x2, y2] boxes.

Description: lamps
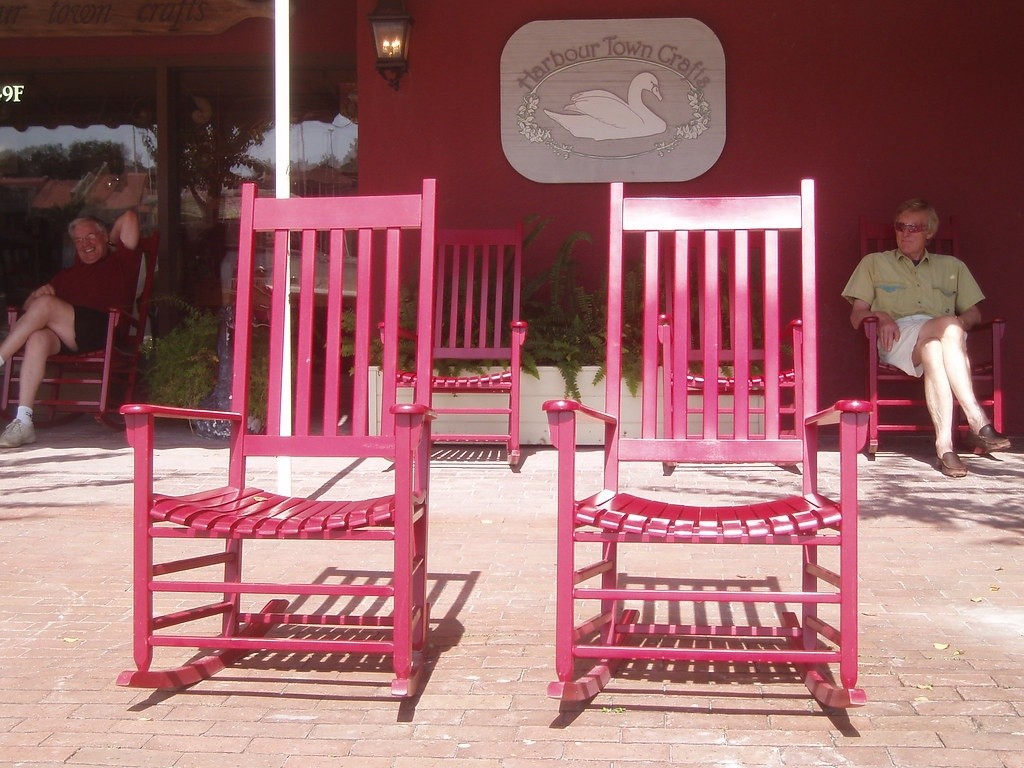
[[368, 0, 416, 92]]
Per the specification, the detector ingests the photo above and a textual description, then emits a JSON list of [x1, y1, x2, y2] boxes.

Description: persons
[[0, 211, 147, 448], [842, 199, 1011, 477]]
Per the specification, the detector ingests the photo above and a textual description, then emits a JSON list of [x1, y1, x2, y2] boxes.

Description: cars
[[144, 186, 357, 360]]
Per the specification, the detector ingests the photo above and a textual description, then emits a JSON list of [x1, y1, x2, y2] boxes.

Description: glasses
[[73, 230, 103, 246], [895, 220, 929, 233]]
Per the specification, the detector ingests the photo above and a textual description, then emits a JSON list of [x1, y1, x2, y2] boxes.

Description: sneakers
[[0, 420, 36, 448]]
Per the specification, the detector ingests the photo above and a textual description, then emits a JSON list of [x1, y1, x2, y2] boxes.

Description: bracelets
[[107, 241, 117, 247]]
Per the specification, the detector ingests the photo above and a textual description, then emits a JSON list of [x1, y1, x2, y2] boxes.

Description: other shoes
[[968, 424, 1011, 452], [935, 451, 966, 477]]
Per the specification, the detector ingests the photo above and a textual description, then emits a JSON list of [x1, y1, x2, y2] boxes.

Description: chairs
[[117, 178, 439, 698], [374, 218, 528, 466], [658, 231, 804, 467], [0, 233, 158, 433], [859, 216, 1007, 454], [541, 178, 874, 706]]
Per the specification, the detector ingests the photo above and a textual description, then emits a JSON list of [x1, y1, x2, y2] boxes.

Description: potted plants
[[328, 215, 765, 450], [137, 293, 298, 441]]
